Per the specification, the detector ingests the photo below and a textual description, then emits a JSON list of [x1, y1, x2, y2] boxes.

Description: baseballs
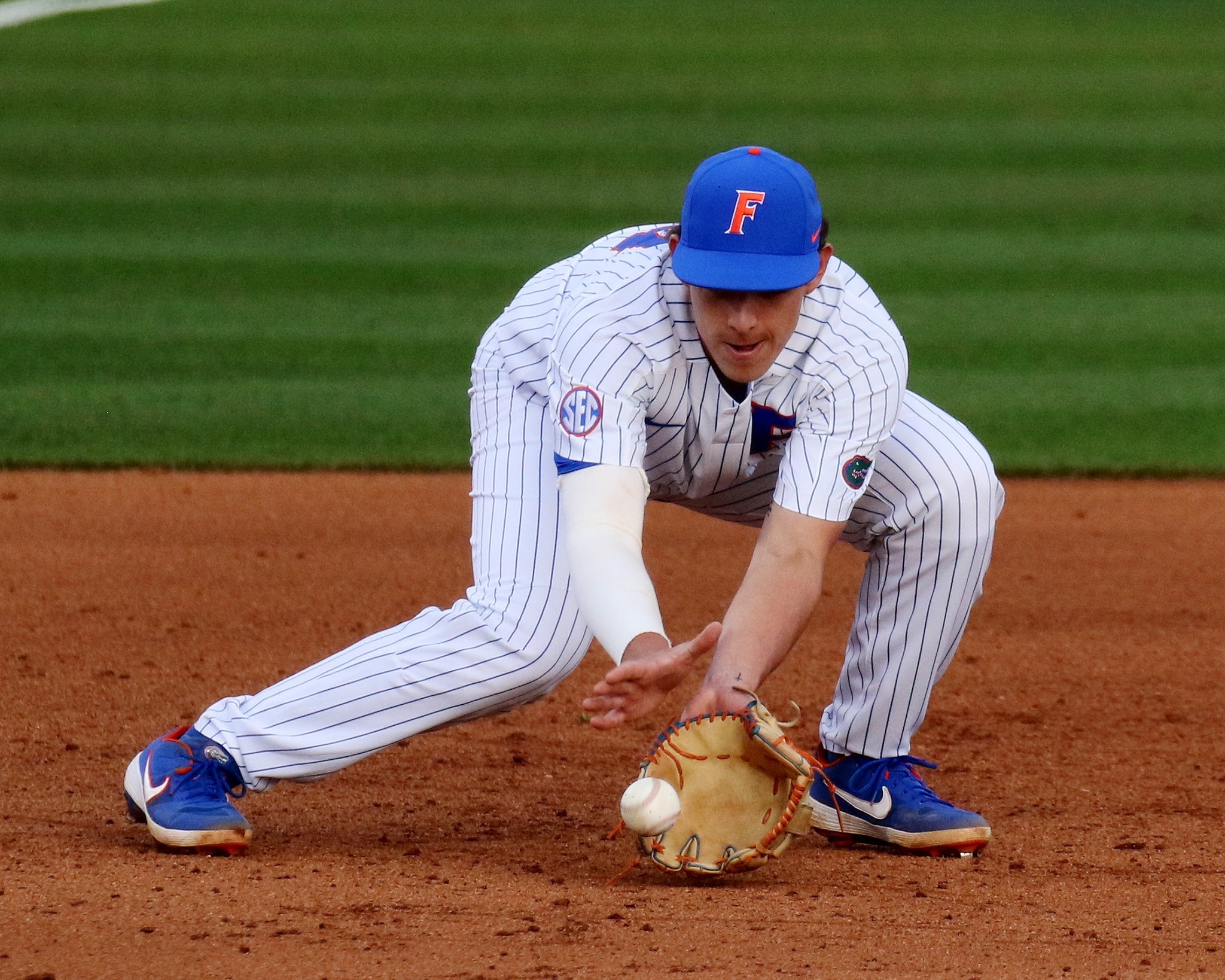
[[618, 778, 681, 838]]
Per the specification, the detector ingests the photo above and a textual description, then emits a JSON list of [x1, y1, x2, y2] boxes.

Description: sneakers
[[124, 725, 253, 855], [804, 742, 992, 858]]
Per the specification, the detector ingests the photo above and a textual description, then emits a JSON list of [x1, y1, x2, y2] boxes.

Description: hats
[[671, 147, 820, 291]]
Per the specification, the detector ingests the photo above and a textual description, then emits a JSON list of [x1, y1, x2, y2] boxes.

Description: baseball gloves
[[634, 700, 815, 876]]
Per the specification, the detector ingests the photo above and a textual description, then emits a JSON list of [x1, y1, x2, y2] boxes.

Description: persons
[[125, 143, 1005, 859]]
